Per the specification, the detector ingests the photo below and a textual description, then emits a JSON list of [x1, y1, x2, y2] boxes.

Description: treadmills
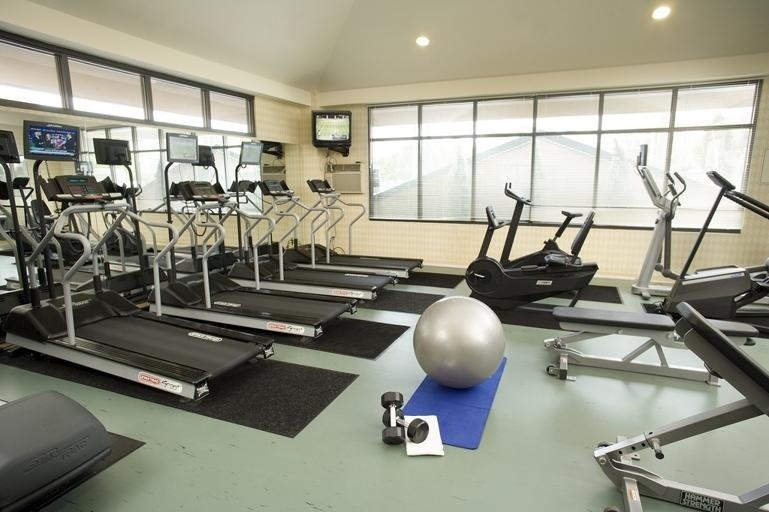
[[0, 131, 424, 402]]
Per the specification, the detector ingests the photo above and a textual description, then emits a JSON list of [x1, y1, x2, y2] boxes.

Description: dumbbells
[[382, 392, 429, 445]]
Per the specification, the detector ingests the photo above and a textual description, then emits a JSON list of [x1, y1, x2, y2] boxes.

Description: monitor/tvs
[[165, 132, 200, 164], [240, 141, 264, 165], [93, 138, 133, 165], [22, 118, 82, 162], [311, 110, 352, 147], [192, 145, 215, 167], [0, 129, 20, 163]]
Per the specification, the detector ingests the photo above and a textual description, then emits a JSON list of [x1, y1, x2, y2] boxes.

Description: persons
[[32, 130, 74, 153]]
